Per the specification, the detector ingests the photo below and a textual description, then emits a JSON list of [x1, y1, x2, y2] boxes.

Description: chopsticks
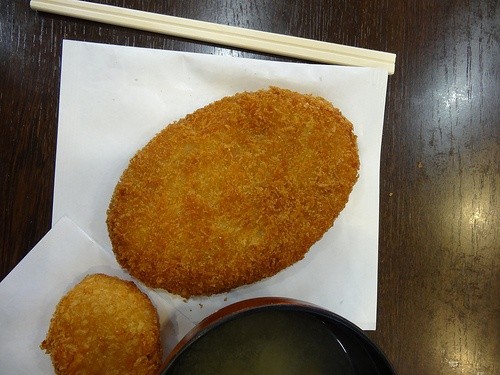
[[30, 0, 397, 76]]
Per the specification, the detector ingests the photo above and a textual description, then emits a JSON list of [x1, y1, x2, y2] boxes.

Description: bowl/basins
[[156, 298, 396, 375]]
